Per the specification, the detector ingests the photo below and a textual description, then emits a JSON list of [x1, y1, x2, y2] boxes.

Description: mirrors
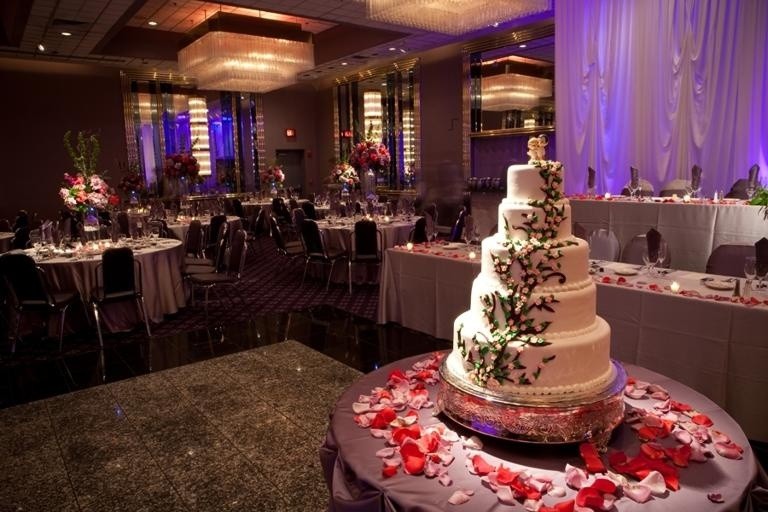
[[119, 69, 265, 197], [331, 56, 423, 198], [461, 17, 556, 192]]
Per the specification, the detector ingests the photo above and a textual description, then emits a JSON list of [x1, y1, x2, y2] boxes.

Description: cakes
[[445, 134, 618, 399]]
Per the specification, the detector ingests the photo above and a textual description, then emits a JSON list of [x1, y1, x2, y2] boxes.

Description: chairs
[[250, 186, 468, 298], [585, 165, 767, 279]]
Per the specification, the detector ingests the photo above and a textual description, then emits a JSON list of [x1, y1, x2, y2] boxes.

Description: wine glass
[[642, 239, 668, 279], [241, 188, 417, 228], [424, 221, 481, 254], [29, 219, 161, 262], [112, 205, 226, 226], [743, 257, 767, 293]]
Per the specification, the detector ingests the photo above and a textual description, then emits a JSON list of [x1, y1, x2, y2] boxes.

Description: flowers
[[348, 139, 391, 172], [117, 162, 145, 191], [58, 129, 120, 212], [165, 134, 201, 180], [323, 155, 359, 186], [261, 159, 286, 185]]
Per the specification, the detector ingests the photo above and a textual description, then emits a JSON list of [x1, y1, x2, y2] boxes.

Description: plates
[[704, 277, 737, 290], [611, 266, 639, 276]]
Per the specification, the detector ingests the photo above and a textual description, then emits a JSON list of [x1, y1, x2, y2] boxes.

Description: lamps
[[367, 1, 556, 40], [188, 93, 212, 178], [478, 55, 554, 111], [178, 6, 314, 95], [401, 104, 416, 175], [362, 89, 382, 144]]
